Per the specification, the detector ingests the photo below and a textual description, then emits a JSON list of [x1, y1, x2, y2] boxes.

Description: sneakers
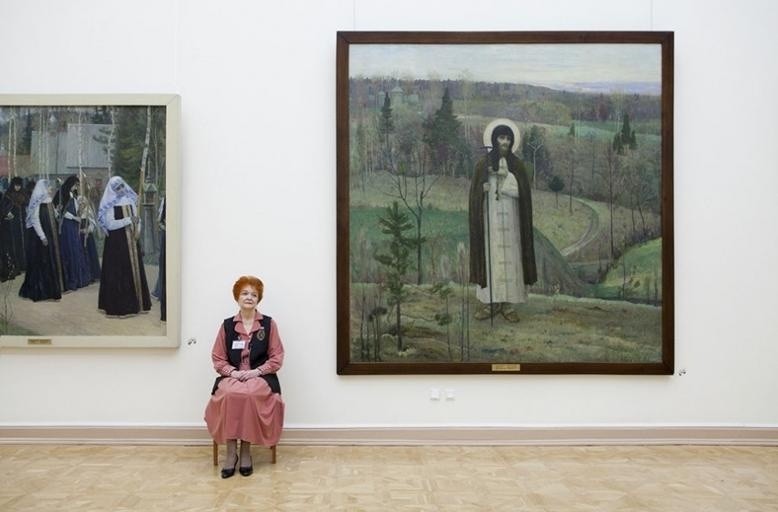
[[501, 301, 520, 322], [474, 303, 502, 320]]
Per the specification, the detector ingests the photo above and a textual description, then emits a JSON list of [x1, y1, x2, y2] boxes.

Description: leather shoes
[[222, 455, 238, 478], [239, 455, 252, 476]]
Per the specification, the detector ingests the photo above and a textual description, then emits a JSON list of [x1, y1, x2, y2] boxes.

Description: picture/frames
[[337, 32, 674, 375], [0, 92, 184, 350]]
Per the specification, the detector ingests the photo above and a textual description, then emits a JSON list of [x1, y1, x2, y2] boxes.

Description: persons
[[466, 118, 537, 325], [149, 195, 166, 300], [96, 174, 153, 317], [1, 171, 103, 305], [205, 275, 286, 478]]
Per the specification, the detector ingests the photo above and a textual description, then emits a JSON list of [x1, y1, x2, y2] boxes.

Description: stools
[[214, 439, 276, 466]]
